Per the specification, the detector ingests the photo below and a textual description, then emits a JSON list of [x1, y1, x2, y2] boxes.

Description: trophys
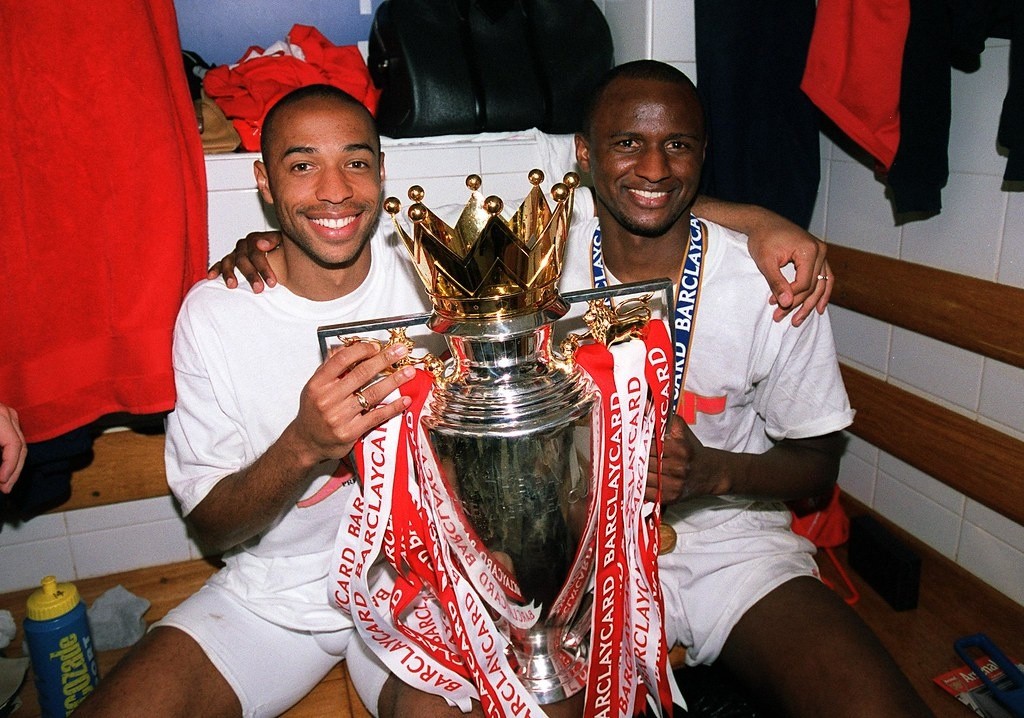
[[338, 168, 651, 703]]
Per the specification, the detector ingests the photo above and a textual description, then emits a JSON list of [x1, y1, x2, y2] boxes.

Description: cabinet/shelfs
[[203, 129, 559, 271]]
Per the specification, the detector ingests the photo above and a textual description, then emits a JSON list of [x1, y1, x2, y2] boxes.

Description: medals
[[656, 523, 677, 556]]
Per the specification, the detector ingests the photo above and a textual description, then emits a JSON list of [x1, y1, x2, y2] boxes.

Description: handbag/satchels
[[365, 0, 614, 139]]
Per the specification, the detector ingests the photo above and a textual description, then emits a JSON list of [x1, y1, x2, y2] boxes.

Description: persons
[[70, 83, 838, 716], [0, 402, 28, 495], [207, 60, 932, 718]]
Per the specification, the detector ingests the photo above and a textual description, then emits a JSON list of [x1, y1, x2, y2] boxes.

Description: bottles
[[22, 575, 101, 717]]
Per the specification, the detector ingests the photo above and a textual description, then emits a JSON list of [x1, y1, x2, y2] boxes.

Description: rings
[[356, 392, 371, 412], [818, 274, 829, 281]]
